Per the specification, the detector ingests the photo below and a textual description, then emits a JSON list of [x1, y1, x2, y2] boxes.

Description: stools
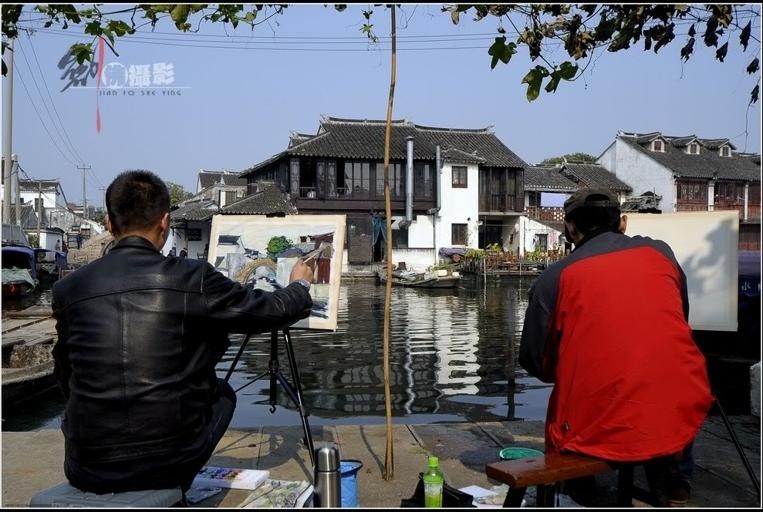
[[30, 481, 184, 506]]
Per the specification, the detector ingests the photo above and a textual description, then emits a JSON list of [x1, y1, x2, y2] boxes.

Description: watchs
[[289, 278, 311, 292]]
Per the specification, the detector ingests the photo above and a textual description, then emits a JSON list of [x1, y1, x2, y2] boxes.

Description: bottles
[[422, 452, 444, 508], [310, 444, 342, 509]]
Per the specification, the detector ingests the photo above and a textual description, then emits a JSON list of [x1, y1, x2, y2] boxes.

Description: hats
[[563, 187, 621, 242]]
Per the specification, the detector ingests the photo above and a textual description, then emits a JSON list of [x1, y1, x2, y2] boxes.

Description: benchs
[[487, 451, 664, 507]]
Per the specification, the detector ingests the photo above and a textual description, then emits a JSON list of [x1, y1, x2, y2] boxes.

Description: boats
[[375, 266, 461, 288], [32, 248, 66, 279], [0, 244, 38, 298]]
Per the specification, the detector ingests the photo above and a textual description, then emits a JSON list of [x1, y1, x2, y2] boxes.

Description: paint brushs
[[303, 246, 327, 263]]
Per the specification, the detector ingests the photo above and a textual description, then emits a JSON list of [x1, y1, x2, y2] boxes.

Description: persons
[[54, 239, 60, 261], [62, 241, 68, 253], [516, 186, 716, 506], [48, 167, 317, 496]]
